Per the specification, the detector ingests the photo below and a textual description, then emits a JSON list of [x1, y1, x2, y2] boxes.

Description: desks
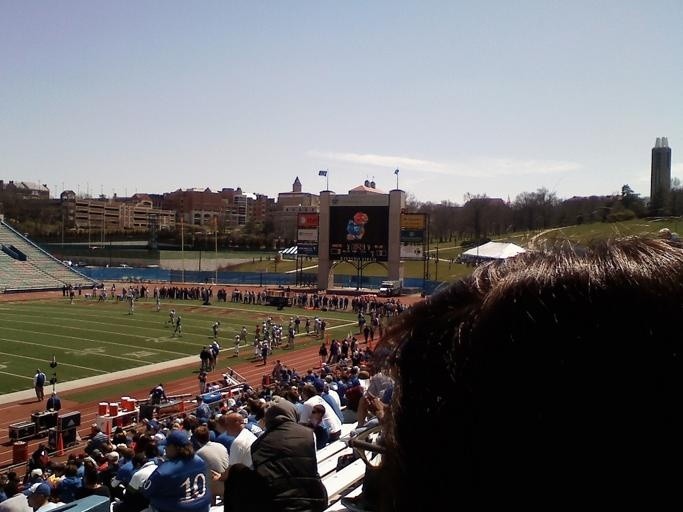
[[96, 408, 140, 434], [156, 399, 184, 416]]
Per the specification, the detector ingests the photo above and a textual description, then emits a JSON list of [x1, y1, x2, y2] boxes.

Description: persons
[[365, 228, 683, 512]]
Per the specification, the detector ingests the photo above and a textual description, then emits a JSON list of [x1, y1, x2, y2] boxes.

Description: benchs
[[211, 427, 383, 512]]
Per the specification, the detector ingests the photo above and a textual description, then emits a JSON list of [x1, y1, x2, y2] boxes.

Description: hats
[[196, 395, 204, 401], [22, 482, 50, 497], [31, 468, 41, 478], [313, 379, 324, 387], [148, 420, 188, 446], [351, 365, 370, 379], [325, 375, 333, 382], [132, 454, 148, 470], [104, 443, 135, 465], [51, 462, 65, 471]]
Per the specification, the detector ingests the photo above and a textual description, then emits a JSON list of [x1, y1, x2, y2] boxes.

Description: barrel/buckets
[[110, 403, 119, 416], [121, 397, 130, 412], [12, 440, 29, 464], [126, 399, 135, 411], [98, 403, 108, 415]]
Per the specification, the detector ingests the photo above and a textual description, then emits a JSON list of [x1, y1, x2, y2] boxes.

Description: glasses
[[312, 411, 318, 413], [349, 424, 399, 472]]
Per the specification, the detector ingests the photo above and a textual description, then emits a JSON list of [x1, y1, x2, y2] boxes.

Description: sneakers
[[341, 492, 363, 512], [338, 454, 355, 467]]
